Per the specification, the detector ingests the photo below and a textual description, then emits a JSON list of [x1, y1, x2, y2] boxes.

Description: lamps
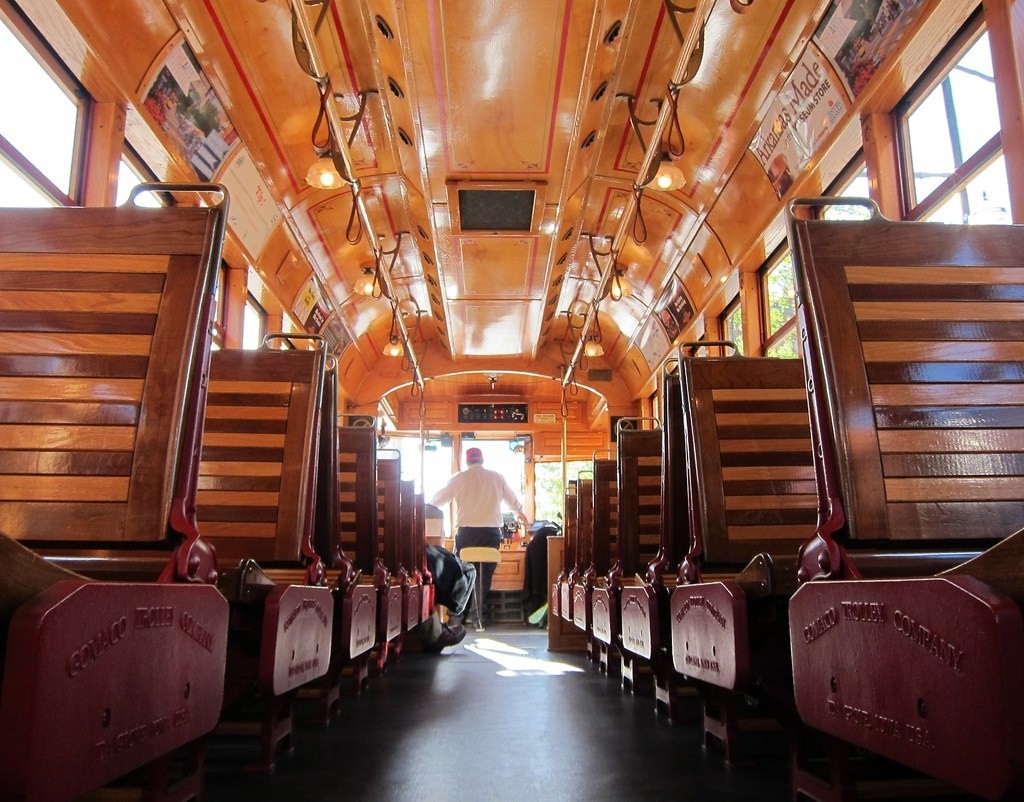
[[648, 143, 687, 191], [306, 140, 346, 190], [607, 264, 632, 295], [355, 264, 380, 296], [583, 331, 604, 357], [383, 333, 404, 357]]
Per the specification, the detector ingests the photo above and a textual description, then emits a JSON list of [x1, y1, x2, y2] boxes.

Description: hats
[[466, 448, 482, 460]]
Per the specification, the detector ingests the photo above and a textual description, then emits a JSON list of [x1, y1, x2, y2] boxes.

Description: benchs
[[551, 195, 1024, 802], [0, 183, 434, 802]]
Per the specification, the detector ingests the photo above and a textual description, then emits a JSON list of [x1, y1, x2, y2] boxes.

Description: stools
[[459, 545, 502, 631]]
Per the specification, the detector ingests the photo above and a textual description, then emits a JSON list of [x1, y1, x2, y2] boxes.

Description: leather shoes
[[433, 625, 466, 653]]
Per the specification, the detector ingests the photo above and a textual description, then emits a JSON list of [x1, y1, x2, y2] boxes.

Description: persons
[[431, 447, 528, 629], [411, 544, 478, 656]]
